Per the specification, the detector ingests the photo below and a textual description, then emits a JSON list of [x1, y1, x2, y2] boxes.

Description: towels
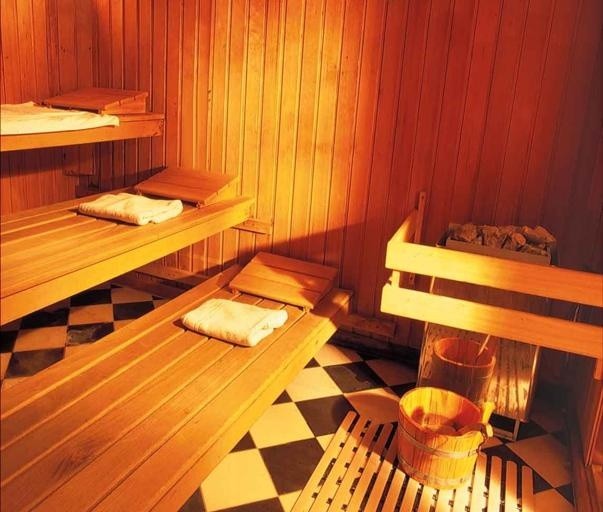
[[0, 102, 119, 136], [76, 192, 183, 226], [183, 299, 290, 347]]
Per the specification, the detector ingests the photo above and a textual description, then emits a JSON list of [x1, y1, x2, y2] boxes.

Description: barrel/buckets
[[432, 337, 496, 416], [399, 388, 485, 489]]
